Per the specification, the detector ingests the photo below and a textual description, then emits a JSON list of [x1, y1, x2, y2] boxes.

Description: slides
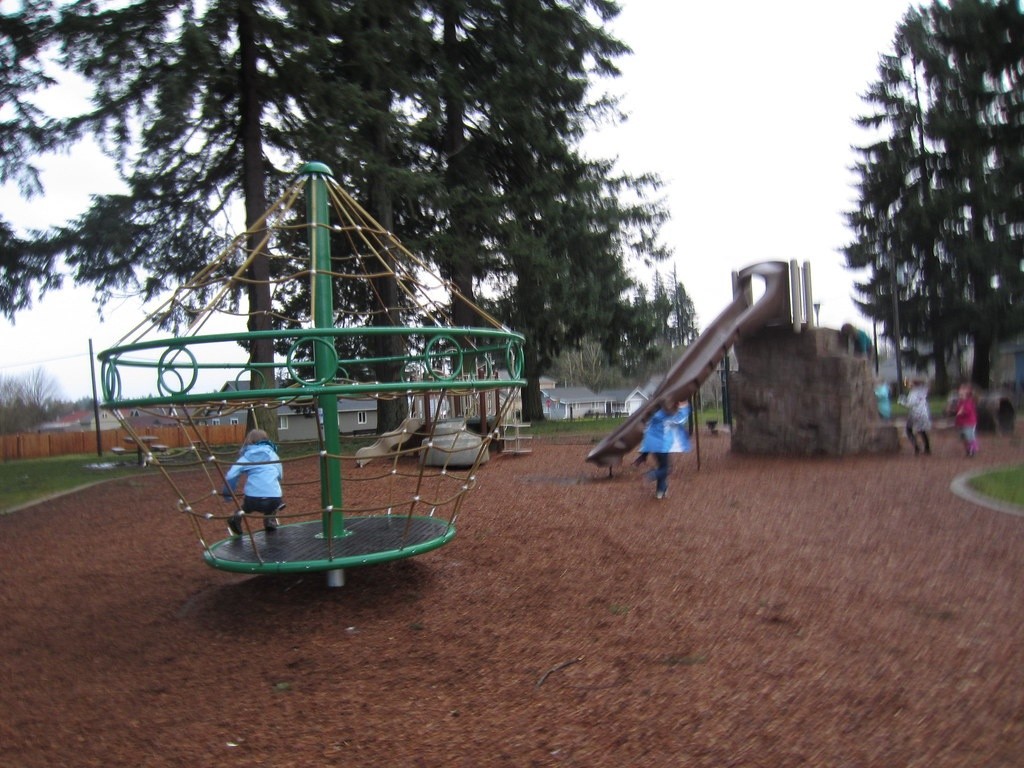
[[354, 418, 425, 468], [584, 296, 777, 469]]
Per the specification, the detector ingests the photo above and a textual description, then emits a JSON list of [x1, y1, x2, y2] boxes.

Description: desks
[[123, 437, 159, 465]]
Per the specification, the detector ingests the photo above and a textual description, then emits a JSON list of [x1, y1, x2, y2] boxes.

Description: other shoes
[[227, 516, 243, 535], [265, 518, 276, 532]]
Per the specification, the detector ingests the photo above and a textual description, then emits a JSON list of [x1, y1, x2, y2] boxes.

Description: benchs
[[112, 447, 126, 455], [151, 443, 168, 452]]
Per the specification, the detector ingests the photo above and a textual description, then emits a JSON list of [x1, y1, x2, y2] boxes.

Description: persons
[[841, 324, 873, 362], [956, 386, 980, 457], [898, 379, 931, 457], [638, 397, 692, 500], [873, 376, 890, 420], [221, 430, 282, 531]]
